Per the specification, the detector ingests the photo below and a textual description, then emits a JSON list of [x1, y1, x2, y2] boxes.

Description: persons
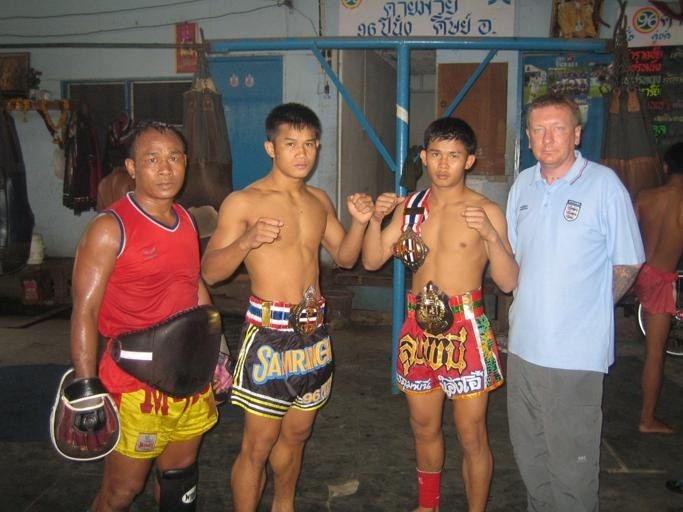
[[631, 141, 683, 434], [94, 142, 136, 211], [507, 94, 647, 512], [362, 118, 520, 512], [62, 122, 230, 512], [200, 101, 374, 512]]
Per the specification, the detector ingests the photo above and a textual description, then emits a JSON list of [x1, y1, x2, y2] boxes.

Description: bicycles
[[635, 271, 683, 358]]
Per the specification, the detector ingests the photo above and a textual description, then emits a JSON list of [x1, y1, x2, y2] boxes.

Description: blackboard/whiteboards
[[624, 45, 683, 158]]
